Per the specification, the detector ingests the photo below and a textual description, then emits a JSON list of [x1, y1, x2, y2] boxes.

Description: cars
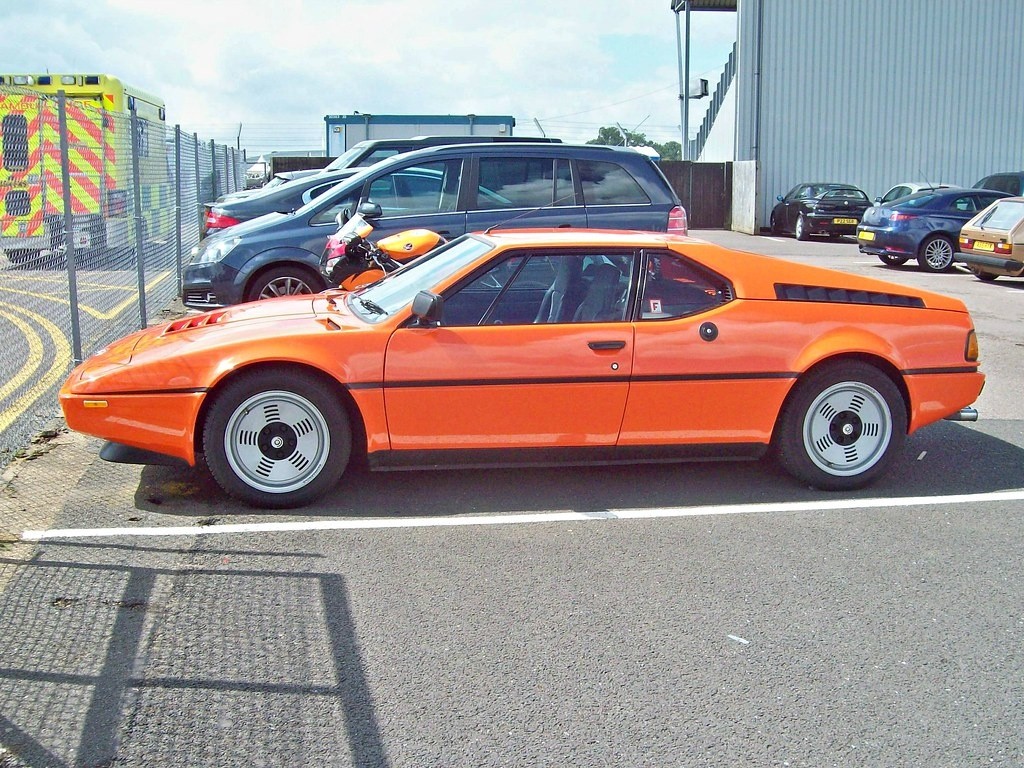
[[873, 182, 979, 213], [199, 166, 512, 244], [951, 194, 1024, 282], [854, 188, 1019, 273], [770, 183, 874, 242]]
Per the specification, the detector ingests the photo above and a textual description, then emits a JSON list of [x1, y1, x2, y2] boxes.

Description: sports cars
[[57, 222, 989, 513]]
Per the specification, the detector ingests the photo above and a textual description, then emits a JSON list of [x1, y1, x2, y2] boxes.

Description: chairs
[[532, 255, 582, 323], [966, 201, 974, 210], [570, 264, 620, 321]]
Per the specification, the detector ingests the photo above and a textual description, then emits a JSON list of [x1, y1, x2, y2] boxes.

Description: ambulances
[[1, 74, 175, 264]]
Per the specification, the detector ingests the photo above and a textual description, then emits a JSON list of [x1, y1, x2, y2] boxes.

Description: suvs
[[176, 140, 689, 315], [969, 171, 1024, 199], [318, 133, 566, 190]]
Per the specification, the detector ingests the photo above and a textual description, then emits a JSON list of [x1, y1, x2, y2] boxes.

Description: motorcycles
[[317, 203, 448, 294]]
